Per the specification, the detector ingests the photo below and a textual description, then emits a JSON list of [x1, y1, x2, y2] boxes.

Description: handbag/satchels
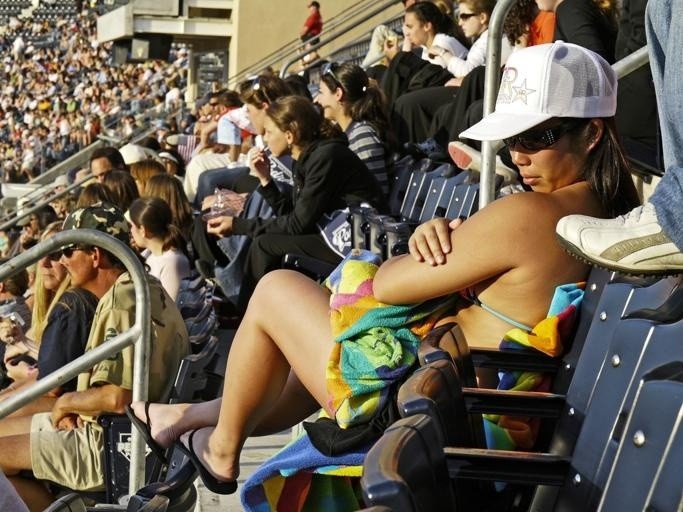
[[320, 203, 373, 259]]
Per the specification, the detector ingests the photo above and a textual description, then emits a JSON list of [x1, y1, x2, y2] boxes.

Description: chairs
[[19, 83, 683, 512]]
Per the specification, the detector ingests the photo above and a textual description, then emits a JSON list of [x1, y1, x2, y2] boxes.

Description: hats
[[458, 41, 618, 143], [119, 143, 145, 166], [50, 203, 131, 253]]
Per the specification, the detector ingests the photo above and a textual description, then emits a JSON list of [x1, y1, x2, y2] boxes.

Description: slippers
[[125, 399, 168, 470], [174, 427, 237, 494]]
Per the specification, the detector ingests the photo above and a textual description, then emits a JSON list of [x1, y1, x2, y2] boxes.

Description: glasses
[[253, 76, 262, 91], [64, 247, 82, 257], [323, 62, 340, 86], [504, 118, 568, 151], [461, 12, 476, 19]]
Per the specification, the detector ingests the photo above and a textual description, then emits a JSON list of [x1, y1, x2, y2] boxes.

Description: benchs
[[0, 1, 76, 55]]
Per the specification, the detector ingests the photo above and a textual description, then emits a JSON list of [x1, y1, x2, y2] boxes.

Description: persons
[[1, 0, 683, 512]]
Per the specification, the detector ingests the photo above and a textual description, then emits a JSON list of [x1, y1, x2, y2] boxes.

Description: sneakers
[[556, 202, 682, 277], [198, 259, 218, 284], [449, 140, 516, 188]]
